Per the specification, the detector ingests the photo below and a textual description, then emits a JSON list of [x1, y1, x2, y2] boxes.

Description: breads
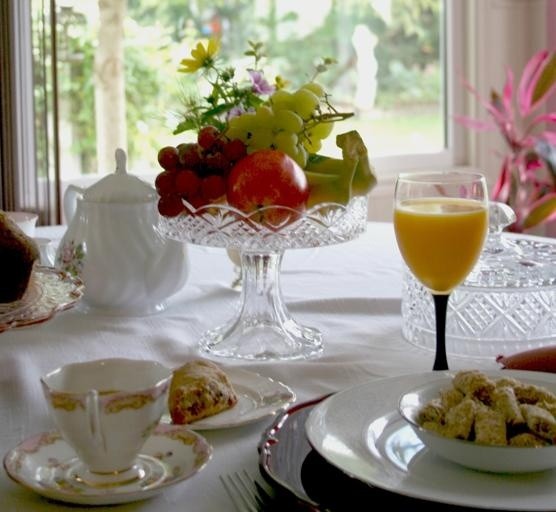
[[168, 359, 238, 424]]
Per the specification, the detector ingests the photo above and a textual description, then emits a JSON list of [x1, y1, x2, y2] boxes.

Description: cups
[[41, 358, 170, 485], [3, 210, 38, 235]]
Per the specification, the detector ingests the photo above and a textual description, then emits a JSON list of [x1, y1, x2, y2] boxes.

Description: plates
[[0, 264, 83, 333], [4, 423, 216, 506], [399, 375, 555, 475], [157, 367, 295, 432], [304, 367, 556, 510]]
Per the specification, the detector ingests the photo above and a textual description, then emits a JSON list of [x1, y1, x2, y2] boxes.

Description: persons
[[501, 344, 555, 374]]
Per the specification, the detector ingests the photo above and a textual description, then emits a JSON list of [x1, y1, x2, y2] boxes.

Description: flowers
[[163, 36, 338, 138]]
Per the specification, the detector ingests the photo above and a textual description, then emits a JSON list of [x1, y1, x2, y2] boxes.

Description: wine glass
[[391, 169, 491, 367], [226, 247, 244, 291]]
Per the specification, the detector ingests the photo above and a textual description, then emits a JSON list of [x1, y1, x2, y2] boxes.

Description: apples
[[227, 147, 310, 232]]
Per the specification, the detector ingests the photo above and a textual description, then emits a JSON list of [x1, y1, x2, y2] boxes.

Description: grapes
[[221, 83, 355, 170]]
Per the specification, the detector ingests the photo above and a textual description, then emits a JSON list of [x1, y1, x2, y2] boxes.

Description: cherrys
[[156, 126, 245, 217]]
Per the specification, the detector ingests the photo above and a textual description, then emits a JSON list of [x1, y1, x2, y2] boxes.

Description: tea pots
[[54, 147, 188, 318]]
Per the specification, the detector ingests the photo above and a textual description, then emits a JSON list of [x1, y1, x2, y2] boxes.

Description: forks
[[216, 467, 285, 512]]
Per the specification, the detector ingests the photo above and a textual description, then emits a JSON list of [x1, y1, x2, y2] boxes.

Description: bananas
[[303, 129, 378, 214]]
